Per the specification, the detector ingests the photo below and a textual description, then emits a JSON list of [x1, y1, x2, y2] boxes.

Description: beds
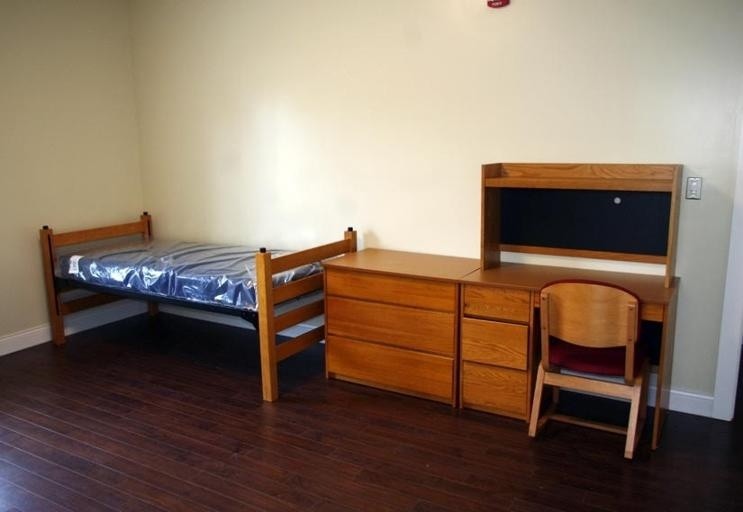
[[38, 211, 357, 404]]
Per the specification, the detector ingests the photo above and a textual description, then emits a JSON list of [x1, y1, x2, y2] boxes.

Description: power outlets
[[685, 176, 701, 201]]
